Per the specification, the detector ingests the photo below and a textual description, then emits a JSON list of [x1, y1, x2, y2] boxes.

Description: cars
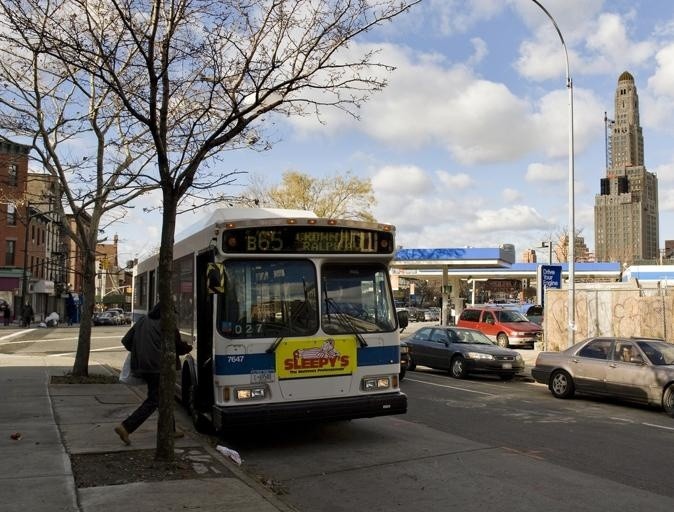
[[404, 326, 524, 381], [92, 304, 132, 326], [389, 291, 543, 327], [399, 339, 409, 383], [455, 305, 543, 347], [531, 337, 673, 417]]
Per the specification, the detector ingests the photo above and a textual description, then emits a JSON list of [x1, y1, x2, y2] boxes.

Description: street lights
[[532, 240, 553, 265], [100, 251, 138, 311]]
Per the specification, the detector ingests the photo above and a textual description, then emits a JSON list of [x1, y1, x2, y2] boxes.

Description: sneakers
[[115, 425, 131, 443], [175, 427, 184, 438]]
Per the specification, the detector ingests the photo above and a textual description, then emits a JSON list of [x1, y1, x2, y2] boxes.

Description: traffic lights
[[98, 259, 103, 270]]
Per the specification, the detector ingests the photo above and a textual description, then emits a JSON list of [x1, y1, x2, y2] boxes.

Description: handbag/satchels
[[119, 352, 142, 385]]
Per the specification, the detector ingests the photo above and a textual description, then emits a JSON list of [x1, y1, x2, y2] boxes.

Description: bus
[[131, 207, 407, 437]]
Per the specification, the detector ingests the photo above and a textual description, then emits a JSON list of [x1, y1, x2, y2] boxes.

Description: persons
[[114, 300, 196, 448], [3, 303, 11, 327], [20, 300, 36, 328], [616, 344, 635, 362], [320, 281, 363, 318]]
